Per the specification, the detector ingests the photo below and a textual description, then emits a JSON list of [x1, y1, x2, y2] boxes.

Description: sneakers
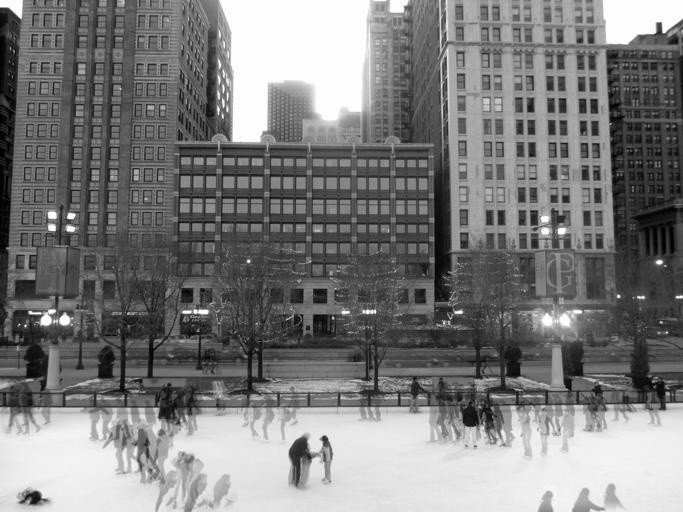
[[288, 478, 333, 489], [464, 438, 498, 448]]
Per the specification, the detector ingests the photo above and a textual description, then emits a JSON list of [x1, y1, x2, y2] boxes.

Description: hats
[[318, 434, 329, 441]]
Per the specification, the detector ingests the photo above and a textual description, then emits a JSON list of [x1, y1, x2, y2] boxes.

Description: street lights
[[183, 303, 206, 369], [536, 204, 571, 394], [38, 201, 78, 392], [73, 301, 92, 374]]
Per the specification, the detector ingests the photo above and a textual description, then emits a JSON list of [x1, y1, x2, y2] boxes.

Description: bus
[[654, 316, 683, 338]]
